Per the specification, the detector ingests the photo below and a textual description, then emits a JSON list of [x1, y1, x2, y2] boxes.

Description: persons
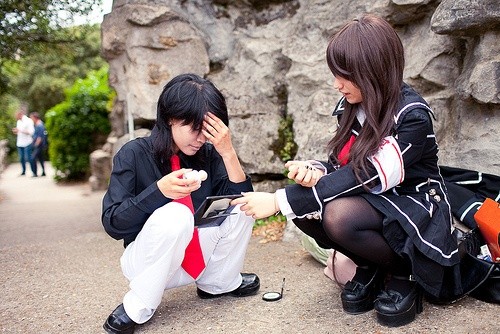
[[30, 112, 47, 176], [229, 15, 496, 326], [12, 110, 35, 176], [101, 73, 260, 334]]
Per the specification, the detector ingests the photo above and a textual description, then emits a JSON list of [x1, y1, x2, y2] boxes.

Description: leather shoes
[[197, 272, 260, 299], [103, 303, 135, 334]]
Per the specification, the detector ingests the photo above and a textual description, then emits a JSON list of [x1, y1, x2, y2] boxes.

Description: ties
[[170, 154, 206, 280], [337, 135, 357, 167]]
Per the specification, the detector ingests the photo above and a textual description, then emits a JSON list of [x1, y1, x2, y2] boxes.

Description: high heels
[[341, 266, 386, 315], [374, 274, 423, 327]]
